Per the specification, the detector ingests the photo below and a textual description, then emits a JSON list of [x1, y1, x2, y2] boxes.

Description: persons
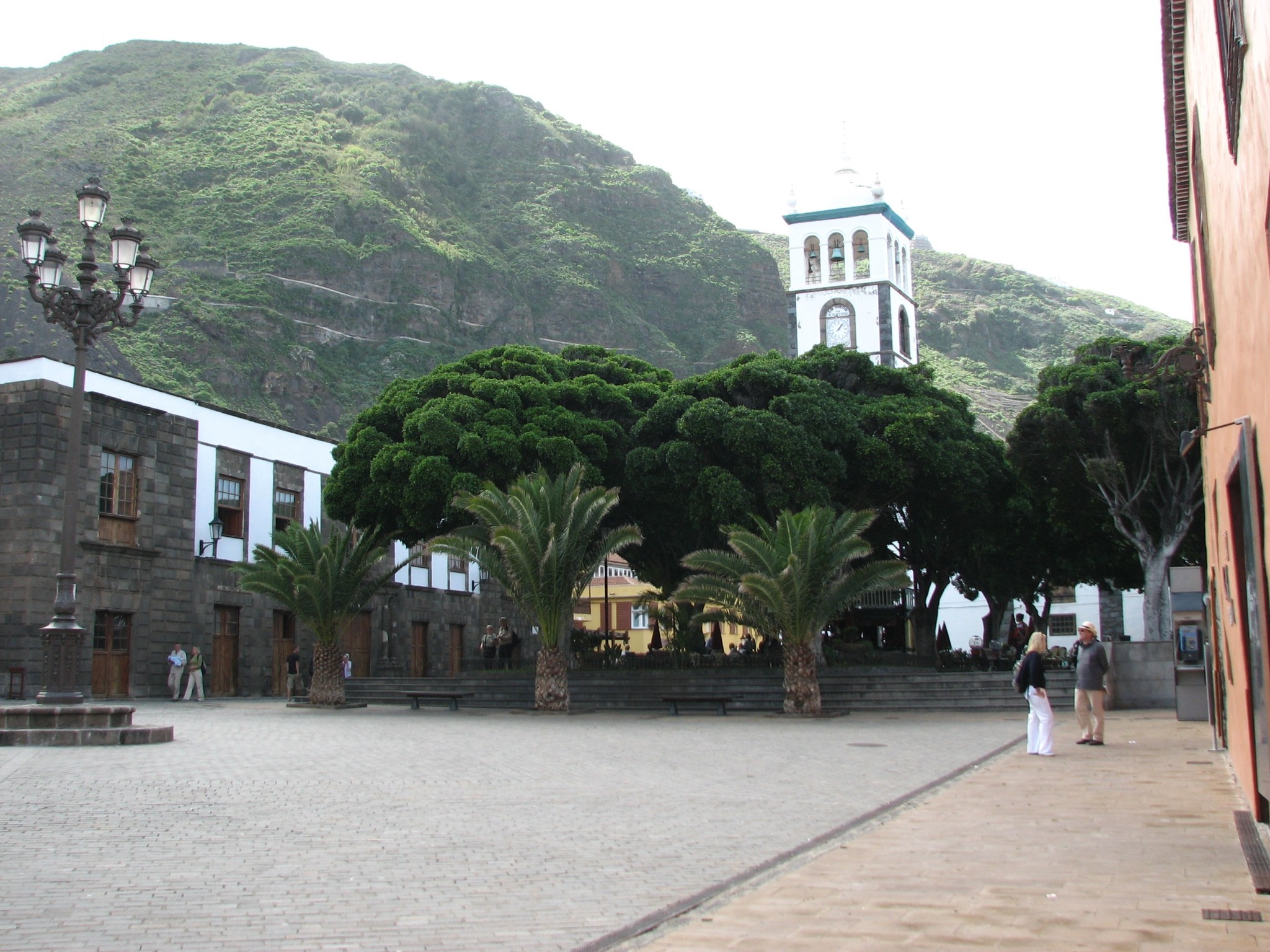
[[286, 645, 304, 702], [167, 642, 205, 702], [309, 656, 314, 684], [342, 654, 352, 677], [705, 627, 779, 660], [1023, 632, 1055, 756], [621, 641, 656, 657], [1010, 609, 1039, 661], [1067, 634, 1113, 669], [1073, 621, 1109, 746], [480, 617, 513, 671]]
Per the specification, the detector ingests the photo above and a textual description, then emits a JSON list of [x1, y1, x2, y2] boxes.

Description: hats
[[1078, 623, 1099, 636]]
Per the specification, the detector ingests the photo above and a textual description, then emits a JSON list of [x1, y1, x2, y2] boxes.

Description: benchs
[[401, 690, 476, 711], [663, 694, 742, 715]]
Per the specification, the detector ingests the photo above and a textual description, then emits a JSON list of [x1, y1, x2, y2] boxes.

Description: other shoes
[[197, 699, 205, 702], [1027, 752, 1040, 755], [1039, 752, 1057, 755], [170, 698, 182, 702], [1075, 738, 1091, 744], [182, 698, 189, 702], [1088, 739, 1105, 745]]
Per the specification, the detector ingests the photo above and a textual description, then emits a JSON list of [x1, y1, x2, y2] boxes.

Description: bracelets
[[1036, 688, 1040, 691]]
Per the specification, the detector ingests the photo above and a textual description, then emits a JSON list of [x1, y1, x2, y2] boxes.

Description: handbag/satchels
[[507, 631, 519, 649], [483, 647, 497, 659], [201, 660, 211, 675], [1012, 654, 1033, 692]]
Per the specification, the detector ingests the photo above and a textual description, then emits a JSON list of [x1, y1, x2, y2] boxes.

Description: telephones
[[1179, 625, 1202, 662]]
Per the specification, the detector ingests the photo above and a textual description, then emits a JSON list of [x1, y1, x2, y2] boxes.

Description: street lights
[[16, 172, 164, 704]]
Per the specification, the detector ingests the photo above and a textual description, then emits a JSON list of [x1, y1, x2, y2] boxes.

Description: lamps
[[471, 564, 489, 592], [1179, 415, 1242, 454], [199, 511, 223, 557]]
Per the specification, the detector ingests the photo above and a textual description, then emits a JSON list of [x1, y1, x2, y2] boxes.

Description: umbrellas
[[937, 622, 952, 652], [711, 620, 723, 653], [652, 618, 663, 650]]
